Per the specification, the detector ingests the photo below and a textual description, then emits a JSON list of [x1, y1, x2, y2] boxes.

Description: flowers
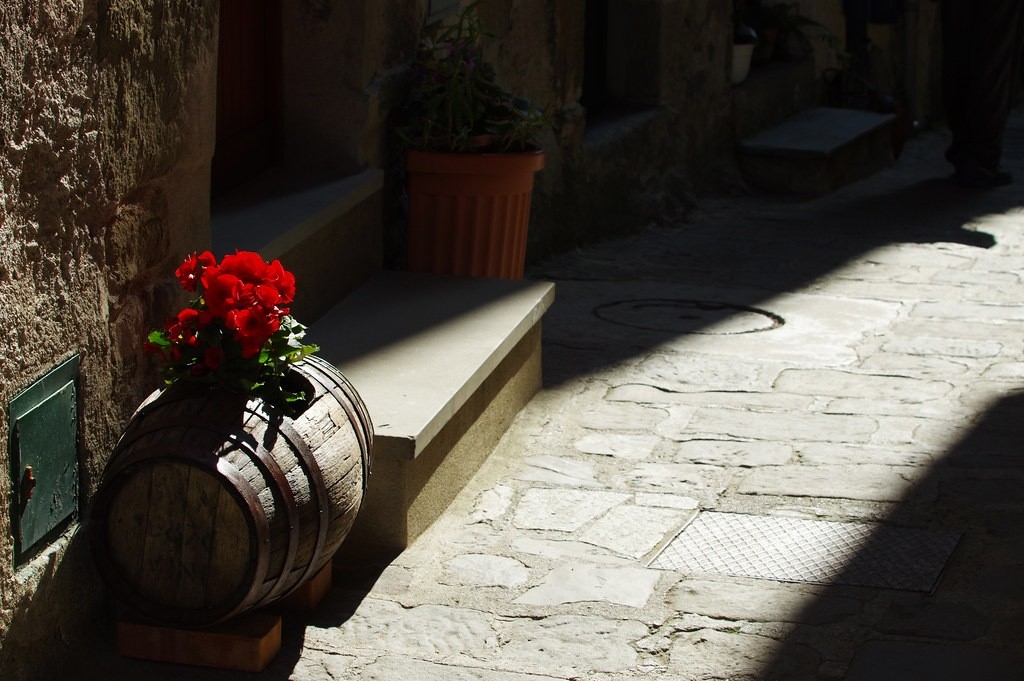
[[388, 0, 558, 165], [137, 247, 318, 404]]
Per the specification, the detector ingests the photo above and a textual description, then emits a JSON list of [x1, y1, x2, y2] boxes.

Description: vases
[[403, 135, 545, 282]]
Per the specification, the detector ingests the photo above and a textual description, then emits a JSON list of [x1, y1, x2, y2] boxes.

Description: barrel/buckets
[[91, 354, 375, 623]]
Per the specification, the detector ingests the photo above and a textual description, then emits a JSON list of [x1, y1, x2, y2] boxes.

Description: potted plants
[[729, 0, 840, 87]]
